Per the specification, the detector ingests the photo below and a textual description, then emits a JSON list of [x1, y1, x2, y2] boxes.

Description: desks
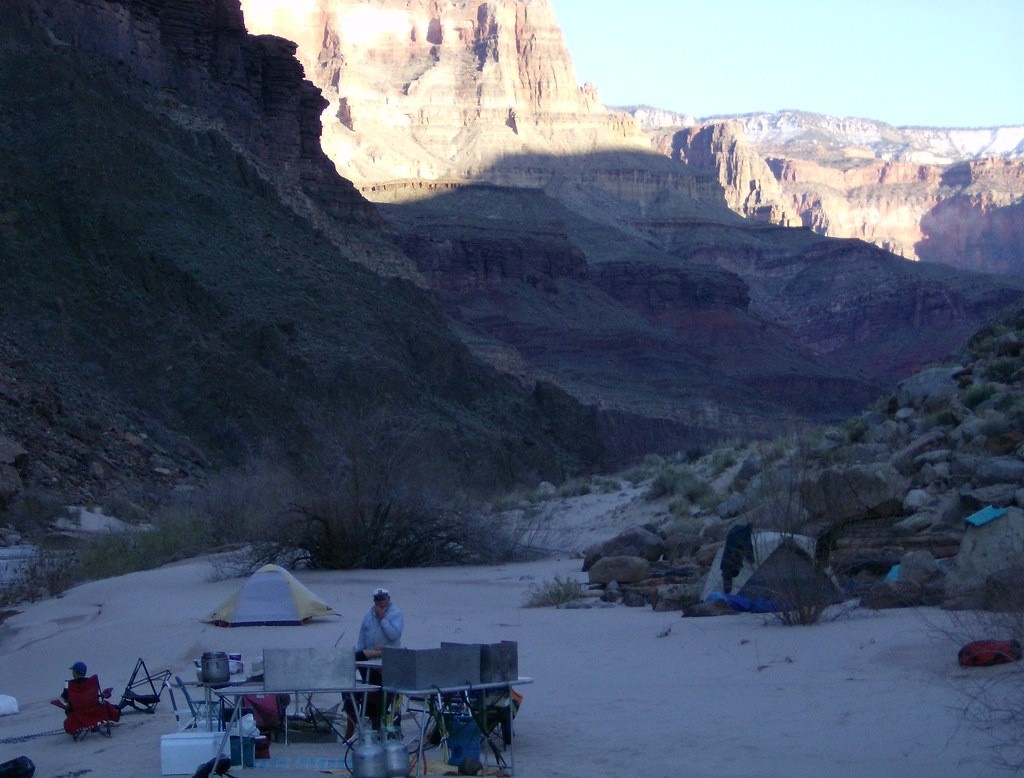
[[167, 658, 534, 778]]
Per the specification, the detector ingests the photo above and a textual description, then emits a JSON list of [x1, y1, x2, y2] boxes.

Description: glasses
[[374, 596, 387, 601]]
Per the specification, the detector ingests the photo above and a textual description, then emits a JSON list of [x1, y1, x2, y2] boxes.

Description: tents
[[200, 564, 343, 625], [734, 538, 843, 612]]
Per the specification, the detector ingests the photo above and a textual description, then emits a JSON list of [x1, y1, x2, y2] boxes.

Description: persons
[[354, 588, 404, 739], [60, 661, 87, 715], [248, 656, 290, 716]]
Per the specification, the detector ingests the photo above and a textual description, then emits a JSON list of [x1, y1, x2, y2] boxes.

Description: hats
[[69, 661, 87, 673], [373, 588, 391, 597]]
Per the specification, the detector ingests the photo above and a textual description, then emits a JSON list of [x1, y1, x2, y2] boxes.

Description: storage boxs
[[160, 732, 231, 776], [230, 735, 254, 767]]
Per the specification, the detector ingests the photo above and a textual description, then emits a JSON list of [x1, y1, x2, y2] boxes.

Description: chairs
[[48, 673, 121, 742], [175, 677, 223, 732], [116, 658, 172, 715]]
